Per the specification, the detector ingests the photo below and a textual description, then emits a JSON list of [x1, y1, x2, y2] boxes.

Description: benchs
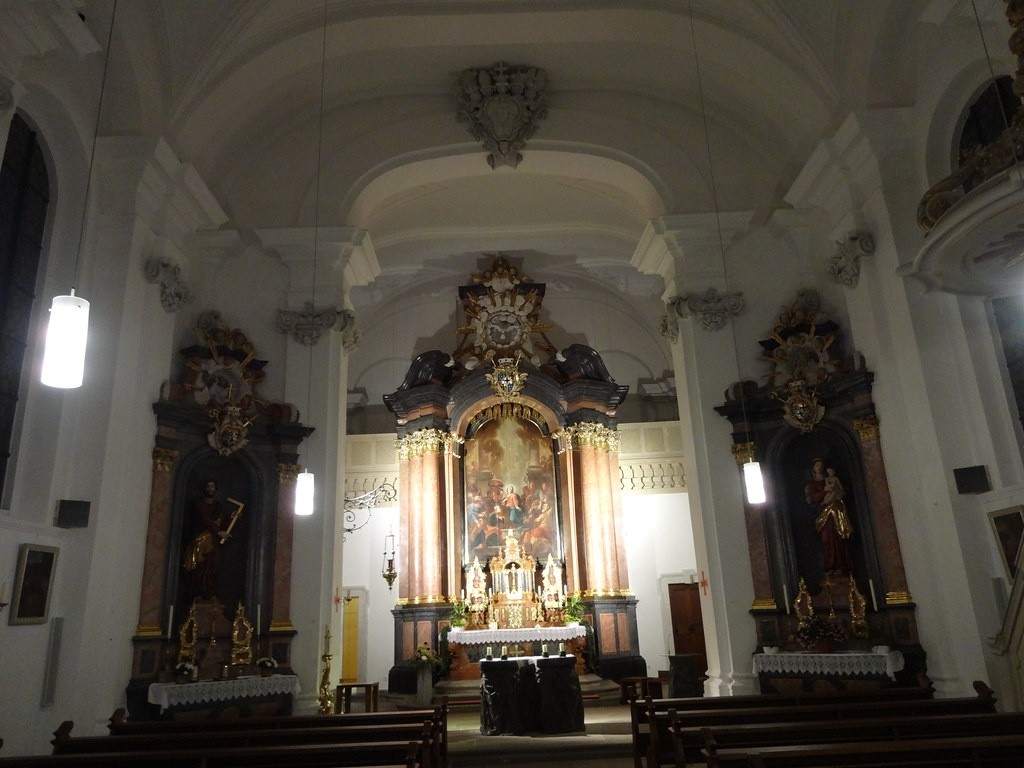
[[0, 694, 448, 768], [625, 681, 1024, 768]]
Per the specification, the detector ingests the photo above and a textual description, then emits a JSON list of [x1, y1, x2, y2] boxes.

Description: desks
[[148, 675, 300, 721], [479, 653, 587, 738], [755, 649, 904, 694], [335, 681, 380, 714], [451, 626, 587, 680]]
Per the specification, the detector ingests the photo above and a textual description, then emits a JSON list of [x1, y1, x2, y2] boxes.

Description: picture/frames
[[8, 543, 60, 625]]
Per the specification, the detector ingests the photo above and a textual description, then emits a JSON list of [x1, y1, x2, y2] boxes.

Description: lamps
[[39, 0, 120, 388], [689, 0, 768, 505], [293, 1, 324, 517]]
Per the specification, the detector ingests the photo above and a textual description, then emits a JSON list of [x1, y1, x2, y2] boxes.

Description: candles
[[502, 645, 507, 655], [257, 605, 261, 636], [538, 585, 542, 596], [559, 642, 565, 652], [542, 645, 548, 653], [489, 588, 492, 597], [564, 585, 567, 595], [486, 647, 492, 654], [461, 589, 465, 599], [783, 584, 790, 615], [868, 579, 878, 611], [168, 606, 174, 638]]
